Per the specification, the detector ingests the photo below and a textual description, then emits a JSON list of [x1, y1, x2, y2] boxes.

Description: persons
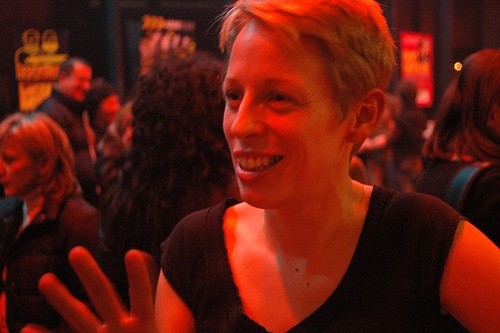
[[0, 0, 500, 333]]
